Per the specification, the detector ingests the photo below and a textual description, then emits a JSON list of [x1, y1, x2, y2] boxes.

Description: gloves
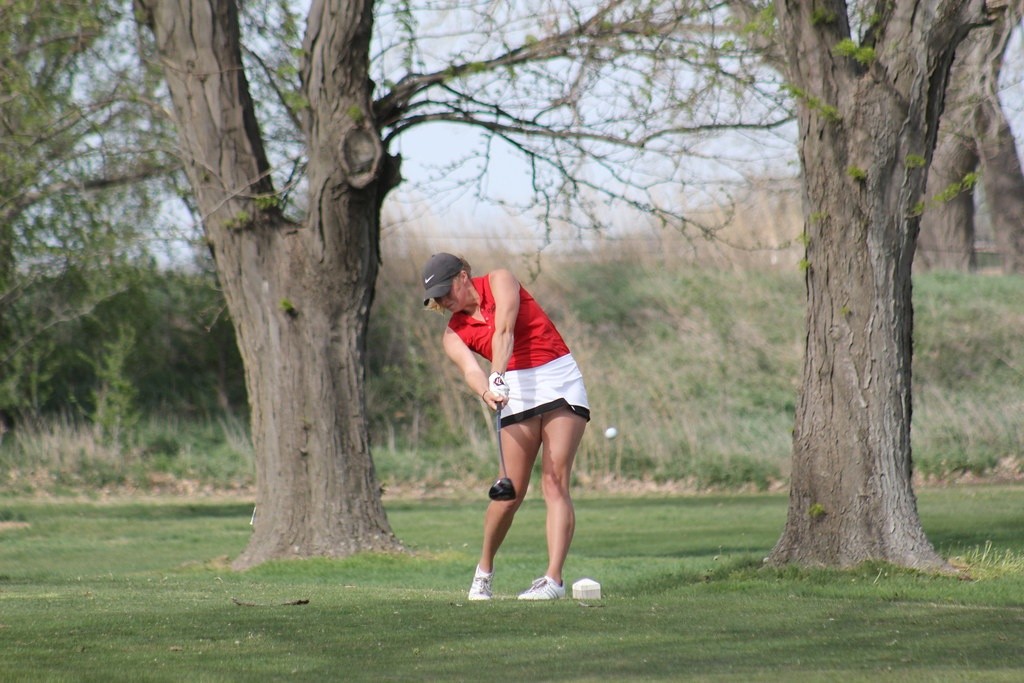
[[487, 371, 510, 398]]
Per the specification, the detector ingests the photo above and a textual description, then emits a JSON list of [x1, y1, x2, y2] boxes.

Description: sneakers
[[469, 564, 494, 600], [517, 577, 565, 600]]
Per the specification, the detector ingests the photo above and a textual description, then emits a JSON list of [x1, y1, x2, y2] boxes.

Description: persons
[[422, 253, 591, 599]]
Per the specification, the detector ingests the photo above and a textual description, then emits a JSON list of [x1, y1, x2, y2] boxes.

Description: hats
[[422, 252, 463, 306]]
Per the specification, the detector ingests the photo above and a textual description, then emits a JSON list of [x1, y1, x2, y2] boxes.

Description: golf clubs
[[488, 403, 517, 501]]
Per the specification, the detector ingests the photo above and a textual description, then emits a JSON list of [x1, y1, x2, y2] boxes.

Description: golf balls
[[605, 427, 618, 440]]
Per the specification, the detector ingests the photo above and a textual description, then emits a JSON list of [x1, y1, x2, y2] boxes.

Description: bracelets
[[483, 391, 487, 402]]
